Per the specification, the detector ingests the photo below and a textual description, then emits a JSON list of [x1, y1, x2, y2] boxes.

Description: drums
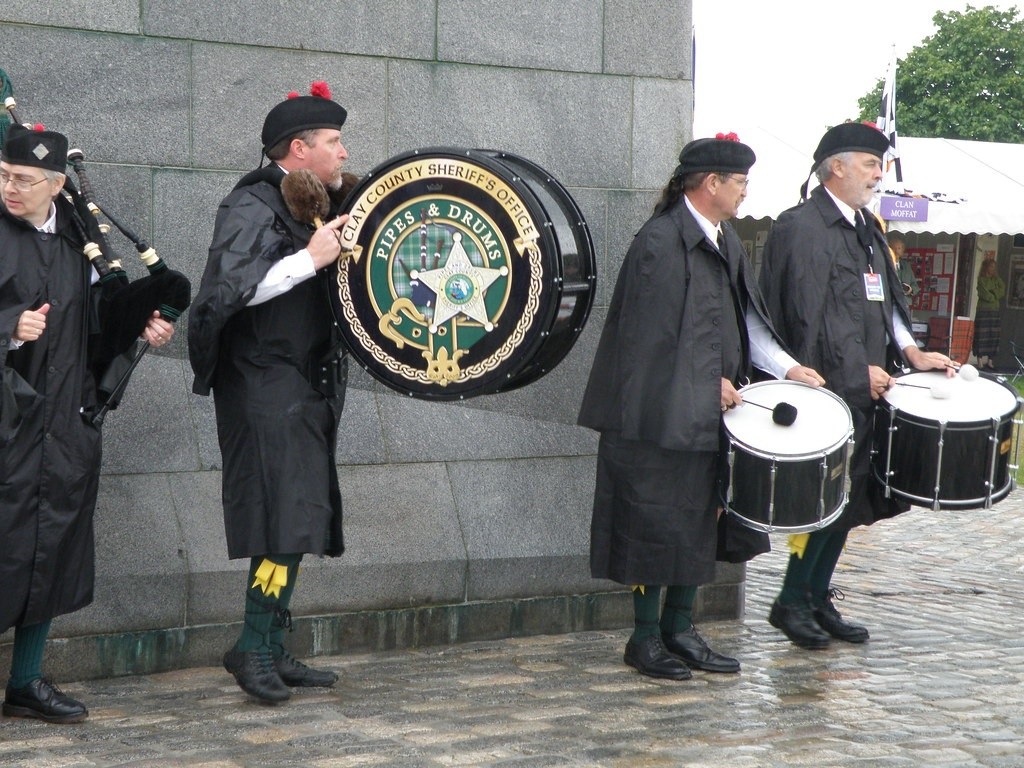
[[871, 368, 1024, 512], [320, 145, 599, 402], [715, 380, 855, 534]]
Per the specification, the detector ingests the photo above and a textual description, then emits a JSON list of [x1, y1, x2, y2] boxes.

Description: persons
[[972, 259, 1006, 369], [0, 124, 175, 720], [889, 239, 921, 308], [757, 123, 958, 650], [187, 82, 359, 700], [575, 134, 826, 680]]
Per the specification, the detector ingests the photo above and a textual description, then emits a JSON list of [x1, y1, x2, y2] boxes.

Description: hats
[[812, 120, 891, 171], [261, 83, 347, 151], [678, 133, 756, 176], [2, 123, 67, 174]]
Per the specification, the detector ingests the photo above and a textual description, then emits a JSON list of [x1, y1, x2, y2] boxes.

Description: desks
[[928, 317, 974, 364]]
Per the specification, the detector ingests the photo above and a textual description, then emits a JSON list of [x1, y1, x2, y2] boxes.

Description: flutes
[[0, 70, 192, 434]]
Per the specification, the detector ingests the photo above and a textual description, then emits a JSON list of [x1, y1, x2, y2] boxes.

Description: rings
[[157, 337, 162, 340]]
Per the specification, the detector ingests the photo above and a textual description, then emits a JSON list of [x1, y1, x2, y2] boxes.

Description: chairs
[[1008, 337, 1024, 383]]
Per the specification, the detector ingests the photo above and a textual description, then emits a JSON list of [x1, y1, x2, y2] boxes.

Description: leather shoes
[[3, 677, 89, 723], [770, 595, 832, 647], [624, 631, 692, 680], [662, 625, 741, 673], [223, 648, 291, 702], [813, 588, 869, 641], [272, 651, 338, 687]]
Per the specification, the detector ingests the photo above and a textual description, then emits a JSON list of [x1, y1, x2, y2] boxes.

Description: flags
[[879, 50, 901, 182]]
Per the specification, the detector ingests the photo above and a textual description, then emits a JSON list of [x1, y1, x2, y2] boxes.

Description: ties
[[854, 210, 871, 247], [718, 230, 729, 261]]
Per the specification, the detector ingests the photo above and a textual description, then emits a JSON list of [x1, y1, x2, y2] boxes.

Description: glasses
[[0, 172, 49, 192]]
